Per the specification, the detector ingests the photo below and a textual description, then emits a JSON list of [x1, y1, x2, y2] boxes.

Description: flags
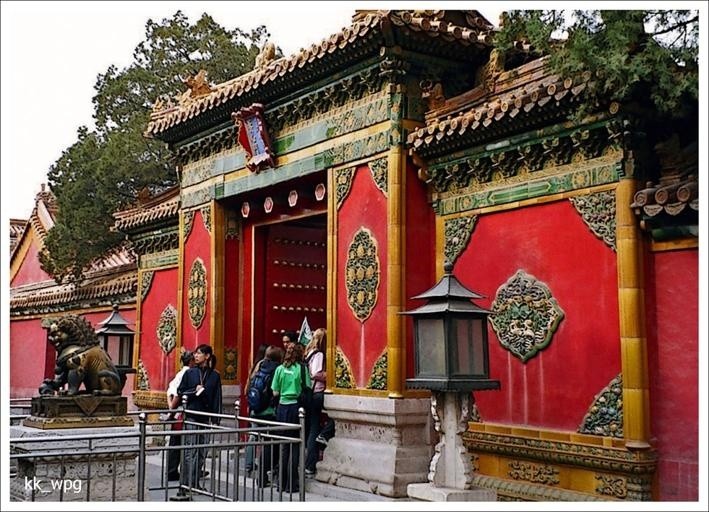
[[297, 316, 312, 347]]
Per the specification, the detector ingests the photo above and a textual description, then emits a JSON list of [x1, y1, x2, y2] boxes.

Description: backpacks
[[245, 360, 276, 413]]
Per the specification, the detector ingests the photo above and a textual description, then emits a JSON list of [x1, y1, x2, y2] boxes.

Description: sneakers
[[316, 433, 328, 446], [304, 467, 315, 479], [168, 468, 210, 495]]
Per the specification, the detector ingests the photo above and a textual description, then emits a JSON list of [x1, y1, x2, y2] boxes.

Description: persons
[[158, 344, 222, 496], [242, 328, 335, 493]]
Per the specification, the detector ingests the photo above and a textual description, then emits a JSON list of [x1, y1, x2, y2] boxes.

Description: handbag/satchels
[[296, 385, 315, 414], [174, 413, 185, 429]]
